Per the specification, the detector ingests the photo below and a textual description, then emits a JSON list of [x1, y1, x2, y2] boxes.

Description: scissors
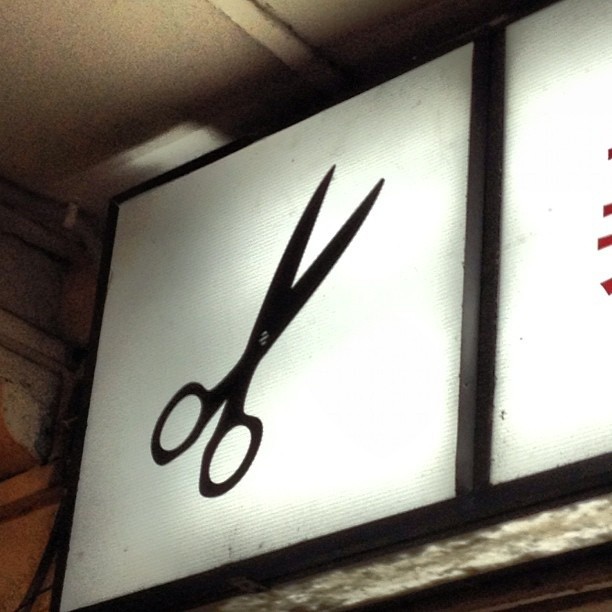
[[150, 164, 384, 498]]
